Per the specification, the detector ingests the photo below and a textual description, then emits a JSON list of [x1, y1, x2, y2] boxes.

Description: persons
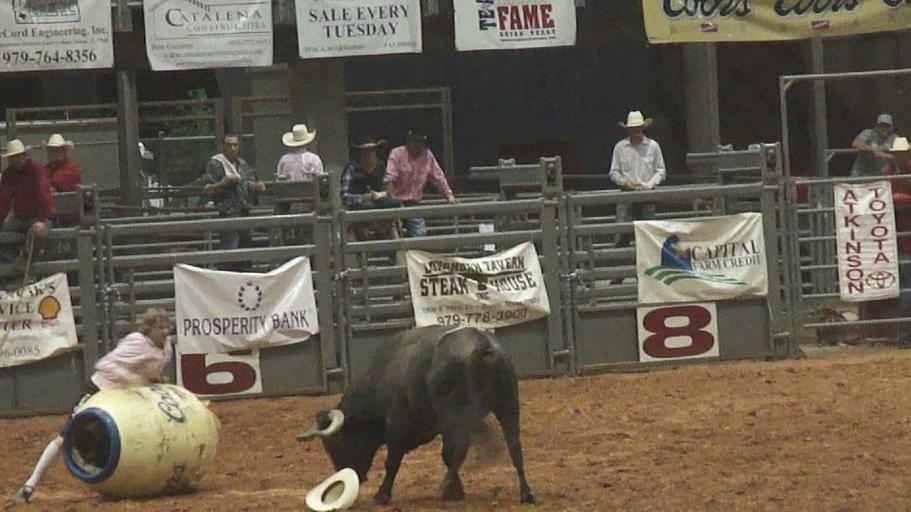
[[0, 139, 55, 280], [851, 115, 897, 179], [40, 135, 82, 285], [384, 125, 460, 296], [881, 138, 911, 253], [608, 109, 666, 286], [201, 131, 266, 271], [275, 124, 324, 244], [18, 308, 174, 504], [340, 131, 403, 301]]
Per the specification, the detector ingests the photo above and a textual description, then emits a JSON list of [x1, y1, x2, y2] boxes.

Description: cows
[[294, 324, 537, 507]]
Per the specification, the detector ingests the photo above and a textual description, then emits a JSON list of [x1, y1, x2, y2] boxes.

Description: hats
[[281, 124, 317, 147], [888, 137, 910, 152], [43, 133, 73, 149], [1, 139, 31, 158], [618, 110, 653, 129], [876, 114, 893, 126], [304, 467, 360, 512], [350, 137, 388, 151]]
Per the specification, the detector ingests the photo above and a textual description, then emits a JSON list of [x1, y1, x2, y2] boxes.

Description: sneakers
[[14, 481, 33, 505]]
